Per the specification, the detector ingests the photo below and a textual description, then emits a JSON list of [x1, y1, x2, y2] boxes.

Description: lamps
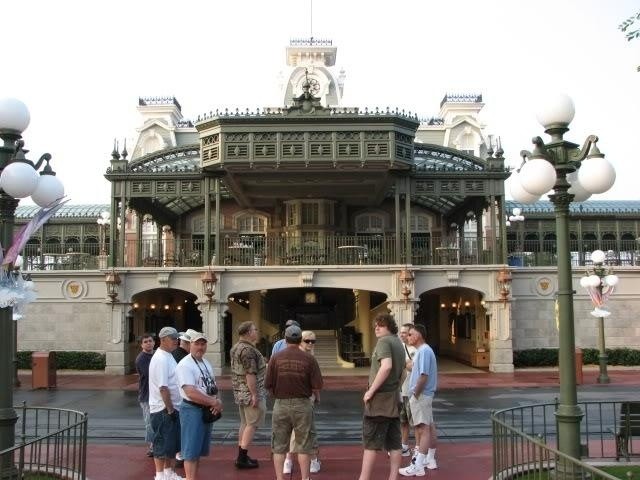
[[201, 268, 218, 308], [497, 267, 513, 301], [104, 268, 123, 304], [396, 266, 414, 306]]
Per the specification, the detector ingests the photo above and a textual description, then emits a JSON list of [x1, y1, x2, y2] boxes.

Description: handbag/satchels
[[201, 406, 221, 424]]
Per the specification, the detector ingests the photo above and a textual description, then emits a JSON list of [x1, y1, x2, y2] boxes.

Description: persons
[[399, 324, 437, 476], [135, 326, 223, 480], [388, 324, 418, 456], [230, 319, 324, 480], [358, 312, 407, 480]]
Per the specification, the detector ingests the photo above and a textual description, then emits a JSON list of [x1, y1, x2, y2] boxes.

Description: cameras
[[208, 384, 219, 395]]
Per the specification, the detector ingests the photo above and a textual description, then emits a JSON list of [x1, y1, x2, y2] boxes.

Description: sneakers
[[388, 448, 438, 477], [147, 447, 186, 480], [310, 461, 321, 473], [235, 456, 259, 469], [282, 463, 292, 474]]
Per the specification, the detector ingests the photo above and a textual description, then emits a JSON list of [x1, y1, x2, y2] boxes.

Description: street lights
[[97, 211, 110, 254], [509, 88, 616, 480], [0, 95, 66, 480], [579, 248, 618, 382], [508, 207, 526, 252], [11, 253, 35, 386]]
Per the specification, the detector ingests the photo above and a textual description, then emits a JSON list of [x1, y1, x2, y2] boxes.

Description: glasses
[[304, 340, 317, 344]]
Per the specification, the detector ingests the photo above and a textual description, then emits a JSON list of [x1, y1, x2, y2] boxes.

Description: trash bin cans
[[31, 351, 57, 391], [575, 346, 583, 387], [507, 256, 524, 266]]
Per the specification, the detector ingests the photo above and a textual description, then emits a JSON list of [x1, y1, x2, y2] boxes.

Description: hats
[[285, 319, 302, 339], [159, 327, 208, 343]]
